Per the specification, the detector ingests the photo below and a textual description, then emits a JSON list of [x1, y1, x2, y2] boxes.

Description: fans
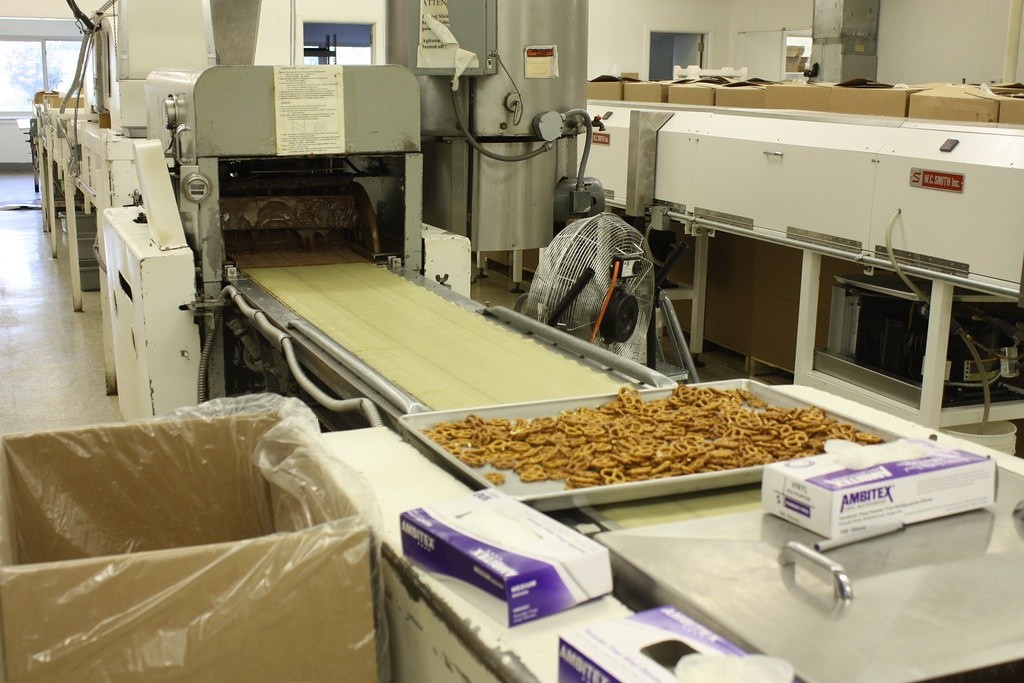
[[525, 212, 654, 359]]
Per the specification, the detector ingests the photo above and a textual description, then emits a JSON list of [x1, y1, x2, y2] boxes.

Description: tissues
[[399, 485, 613, 627], [559, 601, 793, 683], [762, 438, 999, 539]]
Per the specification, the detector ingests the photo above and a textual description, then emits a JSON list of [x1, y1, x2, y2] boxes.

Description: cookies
[[417, 382, 886, 490]]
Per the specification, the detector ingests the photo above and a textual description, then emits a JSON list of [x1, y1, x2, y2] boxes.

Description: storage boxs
[[589, 73, 1023, 125], [0, 413, 383, 683], [400, 488, 614, 627], [34, 91, 84, 108], [786, 45, 808, 73], [558, 606, 799, 683], [761, 436, 998, 540], [485, 209, 863, 373], [58, 212, 101, 292]]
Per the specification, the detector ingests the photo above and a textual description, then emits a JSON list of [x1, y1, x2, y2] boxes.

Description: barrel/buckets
[[943, 421, 1018, 456]]
